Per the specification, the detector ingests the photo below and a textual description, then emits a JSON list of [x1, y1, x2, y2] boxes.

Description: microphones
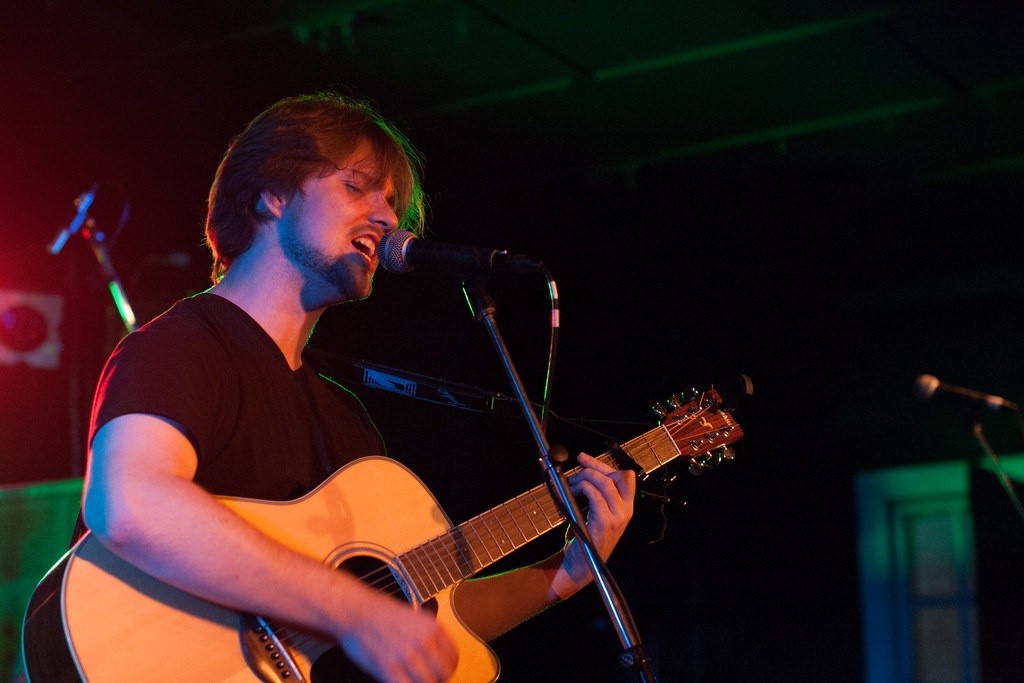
[[48, 187, 97, 253], [376, 229, 543, 273], [918, 375, 1016, 414]]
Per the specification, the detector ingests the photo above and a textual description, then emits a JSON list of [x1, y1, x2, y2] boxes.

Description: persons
[[68, 93, 636, 683]]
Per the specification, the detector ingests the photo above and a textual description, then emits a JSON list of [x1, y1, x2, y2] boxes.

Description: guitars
[[20, 372, 746, 683]]
